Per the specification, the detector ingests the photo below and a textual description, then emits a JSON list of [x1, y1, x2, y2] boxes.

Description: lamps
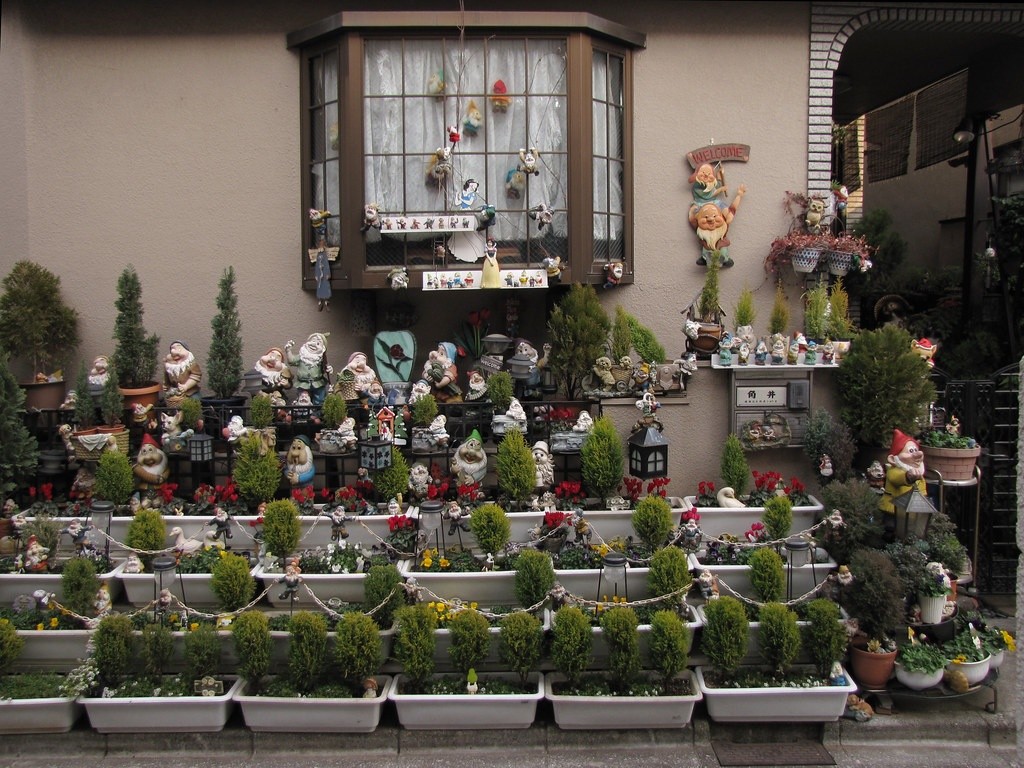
[[151, 556, 179, 591], [600, 553, 627, 584], [952, 113, 976, 144], [90, 500, 116, 572], [419, 501, 446, 530], [784, 537, 812, 568]]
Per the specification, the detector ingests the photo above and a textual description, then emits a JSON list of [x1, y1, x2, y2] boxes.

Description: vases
[[943, 651, 993, 686], [988, 648, 1005, 669], [893, 660, 945, 691]]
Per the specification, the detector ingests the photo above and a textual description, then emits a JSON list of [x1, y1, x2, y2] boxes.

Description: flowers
[[646, 475, 671, 499], [985, 626, 1017, 656], [425, 462, 452, 504], [290, 485, 316, 515], [456, 482, 482, 512], [504, 296, 522, 345], [383, 514, 417, 552], [744, 522, 772, 545], [593, 592, 627, 624], [427, 599, 478, 630], [194, 541, 228, 571], [617, 476, 643, 510], [340, 484, 367, 514], [216, 476, 240, 515], [590, 542, 608, 562], [543, 511, 576, 536], [320, 488, 336, 508], [419, 547, 450, 572], [248, 516, 266, 539], [785, 476, 809, 506], [681, 506, 701, 527], [155, 482, 183, 514], [943, 630, 991, 665], [353, 479, 376, 502], [897, 626, 950, 675], [28, 483, 93, 518], [553, 480, 587, 510], [542, 405, 580, 433], [192, 482, 216, 515], [694, 479, 718, 506], [450, 306, 493, 359], [747, 470, 782, 508]]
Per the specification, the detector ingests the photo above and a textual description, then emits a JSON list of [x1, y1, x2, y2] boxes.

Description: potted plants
[[858, 179, 1022, 391], [916, 515, 967, 625], [0, 263, 897, 735], [916, 427, 982, 481]]
